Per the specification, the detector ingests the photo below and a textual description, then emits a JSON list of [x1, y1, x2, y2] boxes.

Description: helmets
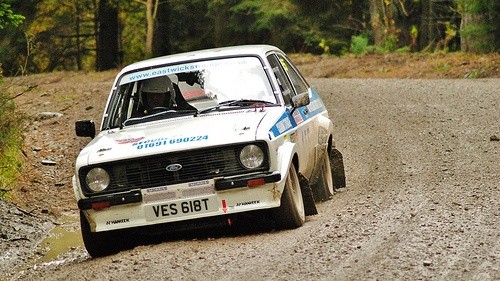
[[139, 75, 177, 110]]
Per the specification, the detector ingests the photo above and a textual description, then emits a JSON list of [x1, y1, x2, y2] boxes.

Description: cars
[[72, 44, 347, 258]]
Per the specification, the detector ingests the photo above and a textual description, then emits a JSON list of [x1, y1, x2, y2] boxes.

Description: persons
[[127, 75, 197, 124]]
